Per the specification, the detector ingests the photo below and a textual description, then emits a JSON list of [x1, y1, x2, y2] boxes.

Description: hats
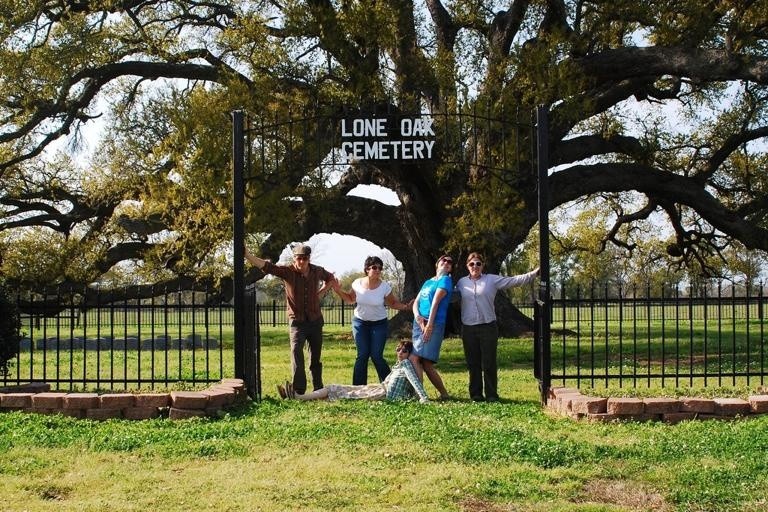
[[292, 245, 311, 257]]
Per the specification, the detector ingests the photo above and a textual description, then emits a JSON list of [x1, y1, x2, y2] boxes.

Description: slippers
[[284, 380, 293, 400], [277, 384, 286, 401]]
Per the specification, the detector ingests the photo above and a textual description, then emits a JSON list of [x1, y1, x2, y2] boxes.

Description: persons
[[452, 252, 539, 403], [243, 240, 334, 394], [407, 254, 458, 400], [277, 339, 431, 404], [331, 256, 414, 385]]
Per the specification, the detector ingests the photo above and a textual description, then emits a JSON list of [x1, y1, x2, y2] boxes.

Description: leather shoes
[[471, 395, 483, 401], [486, 397, 498, 403]]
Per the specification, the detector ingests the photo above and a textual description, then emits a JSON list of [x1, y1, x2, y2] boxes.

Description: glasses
[[441, 258, 452, 265], [468, 262, 481, 267], [371, 265, 382, 271], [396, 349, 407, 353]]
